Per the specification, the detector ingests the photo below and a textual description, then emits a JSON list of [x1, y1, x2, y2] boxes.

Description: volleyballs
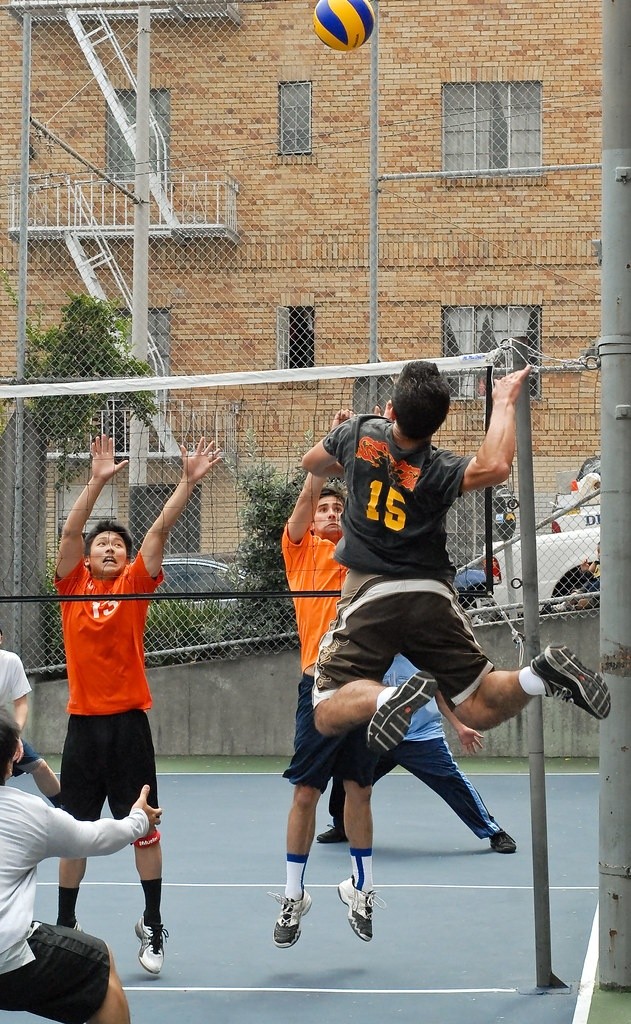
[[312, 0, 377, 52]]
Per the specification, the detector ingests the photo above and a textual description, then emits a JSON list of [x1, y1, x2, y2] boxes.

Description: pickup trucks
[[481, 524, 601, 616], [550, 456, 600, 532]]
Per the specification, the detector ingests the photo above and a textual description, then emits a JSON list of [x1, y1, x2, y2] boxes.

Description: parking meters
[[494, 488, 519, 619]]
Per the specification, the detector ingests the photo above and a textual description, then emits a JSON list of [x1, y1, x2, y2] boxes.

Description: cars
[[146, 556, 266, 620]]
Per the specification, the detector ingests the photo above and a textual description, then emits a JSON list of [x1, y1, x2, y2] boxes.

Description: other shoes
[[490, 828, 516, 853], [317, 829, 347, 842], [551, 602, 566, 611], [575, 599, 589, 610]]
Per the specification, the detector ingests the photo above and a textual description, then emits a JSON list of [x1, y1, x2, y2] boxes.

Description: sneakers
[[135, 912, 164, 974], [531, 643, 611, 720], [74, 915, 82, 931], [365, 671, 438, 754], [338, 875, 376, 942], [273, 889, 312, 948]]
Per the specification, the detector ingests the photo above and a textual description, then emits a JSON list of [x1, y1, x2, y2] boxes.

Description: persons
[[0, 630, 63, 814], [316, 646, 520, 854], [302, 361, 610, 753], [551, 541, 601, 613], [0, 708, 162, 1024], [271, 400, 399, 949], [55, 434, 222, 974]]
